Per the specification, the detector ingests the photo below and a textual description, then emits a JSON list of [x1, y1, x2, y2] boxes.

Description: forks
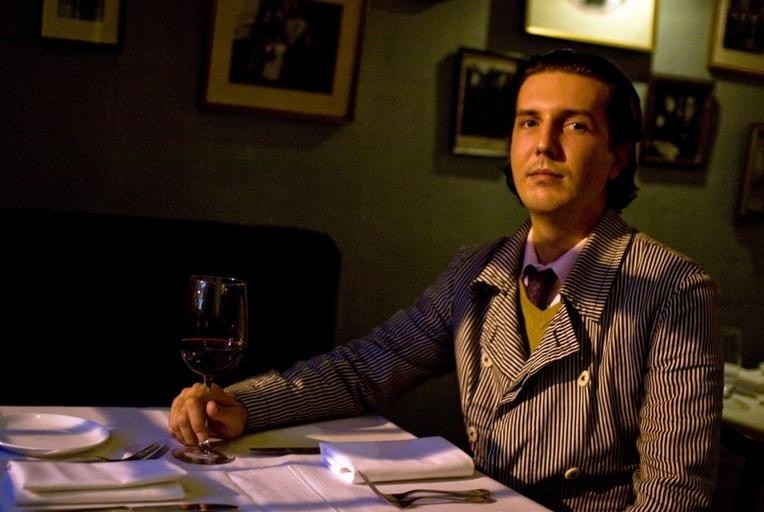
[[356, 469, 497, 510], [71, 441, 168, 463]]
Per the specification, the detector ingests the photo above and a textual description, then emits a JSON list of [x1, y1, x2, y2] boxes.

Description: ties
[[523, 265, 557, 309]]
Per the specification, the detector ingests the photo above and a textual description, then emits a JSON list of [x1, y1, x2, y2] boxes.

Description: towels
[[313, 436, 475, 487], [3, 459, 191, 508], [724, 362, 764, 393]]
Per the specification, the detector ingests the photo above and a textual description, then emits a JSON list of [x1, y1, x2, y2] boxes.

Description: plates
[[0, 413, 110, 459]]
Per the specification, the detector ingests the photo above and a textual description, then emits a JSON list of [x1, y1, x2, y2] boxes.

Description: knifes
[[35, 503, 241, 512], [250, 444, 321, 455]]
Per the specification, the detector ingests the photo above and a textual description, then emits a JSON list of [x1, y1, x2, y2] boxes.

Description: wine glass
[[171, 275, 248, 466]]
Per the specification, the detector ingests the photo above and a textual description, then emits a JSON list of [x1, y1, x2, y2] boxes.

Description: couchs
[[0, 207, 343, 408]]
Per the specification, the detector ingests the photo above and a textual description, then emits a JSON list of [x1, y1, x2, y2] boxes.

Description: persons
[[169, 48, 724, 512]]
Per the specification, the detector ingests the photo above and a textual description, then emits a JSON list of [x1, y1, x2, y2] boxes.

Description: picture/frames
[[449, 43, 531, 159], [516, 1, 659, 75], [638, 72, 716, 170], [732, 120, 764, 229], [706, 0, 760, 80], [30, 0, 128, 59], [195, 0, 371, 127]]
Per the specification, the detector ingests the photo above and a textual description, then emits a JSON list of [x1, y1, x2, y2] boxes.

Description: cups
[[722, 326, 744, 400]]
